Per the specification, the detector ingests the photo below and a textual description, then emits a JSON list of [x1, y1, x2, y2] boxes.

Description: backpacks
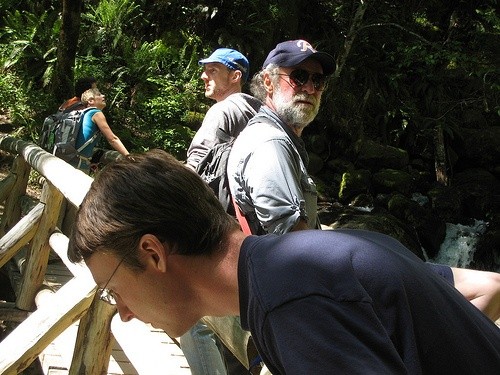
[[37, 106, 101, 169], [192, 115, 293, 236]]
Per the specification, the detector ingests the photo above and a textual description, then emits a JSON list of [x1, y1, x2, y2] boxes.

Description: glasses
[[274, 70, 330, 92], [96, 238, 165, 305]]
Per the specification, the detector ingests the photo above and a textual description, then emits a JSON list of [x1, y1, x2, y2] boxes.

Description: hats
[[198, 48, 250, 82], [262, 40, 336, 76]]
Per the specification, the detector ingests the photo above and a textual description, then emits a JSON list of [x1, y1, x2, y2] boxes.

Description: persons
[[54, 88, 134, 176], [68, 147, 500, 375], [57, 77, 98, 120], [179, 38, 337, 375], [173, 47, 262, 195]]
